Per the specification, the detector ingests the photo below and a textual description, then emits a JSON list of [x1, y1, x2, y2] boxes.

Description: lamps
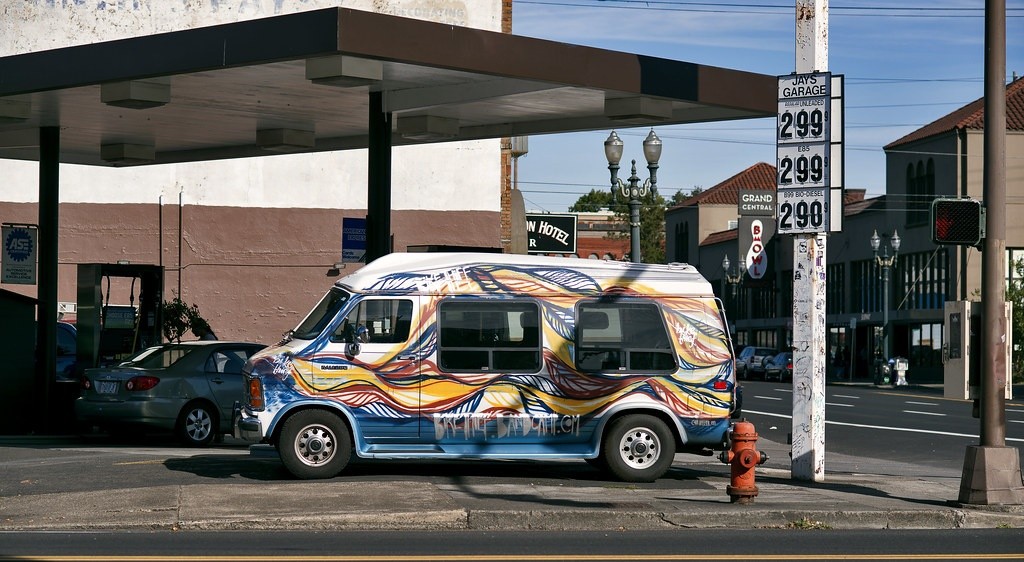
[[333, 264, 346, 269], [117, 261, 130, 264]]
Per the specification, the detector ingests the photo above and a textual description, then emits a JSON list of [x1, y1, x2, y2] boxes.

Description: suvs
[[735, 346, 778, 381]]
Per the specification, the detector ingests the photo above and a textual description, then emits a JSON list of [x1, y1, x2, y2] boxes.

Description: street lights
[[869, 228, 902, 383], [721, 252, 747, 357], [604, 126, 664, 263]]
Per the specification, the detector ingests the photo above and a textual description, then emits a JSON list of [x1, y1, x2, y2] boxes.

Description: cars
[[71, 340, 270, 448], [763, 351, 794, 383]]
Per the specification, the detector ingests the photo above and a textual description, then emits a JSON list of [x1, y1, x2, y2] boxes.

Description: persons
[[190, 317, 220, 370], [57, 312, 64, 321]]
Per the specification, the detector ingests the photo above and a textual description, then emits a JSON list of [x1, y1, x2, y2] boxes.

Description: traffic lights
[[931, 198, 981, 245]]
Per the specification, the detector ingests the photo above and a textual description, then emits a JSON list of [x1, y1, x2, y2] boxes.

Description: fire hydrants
[[716, 415, 771, 508]]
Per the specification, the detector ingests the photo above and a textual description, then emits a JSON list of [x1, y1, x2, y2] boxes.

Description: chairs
[[224, 360, 242, 373]]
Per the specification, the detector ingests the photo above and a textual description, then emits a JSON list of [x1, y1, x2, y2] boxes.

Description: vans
[[231, 251, 740, 483]]
